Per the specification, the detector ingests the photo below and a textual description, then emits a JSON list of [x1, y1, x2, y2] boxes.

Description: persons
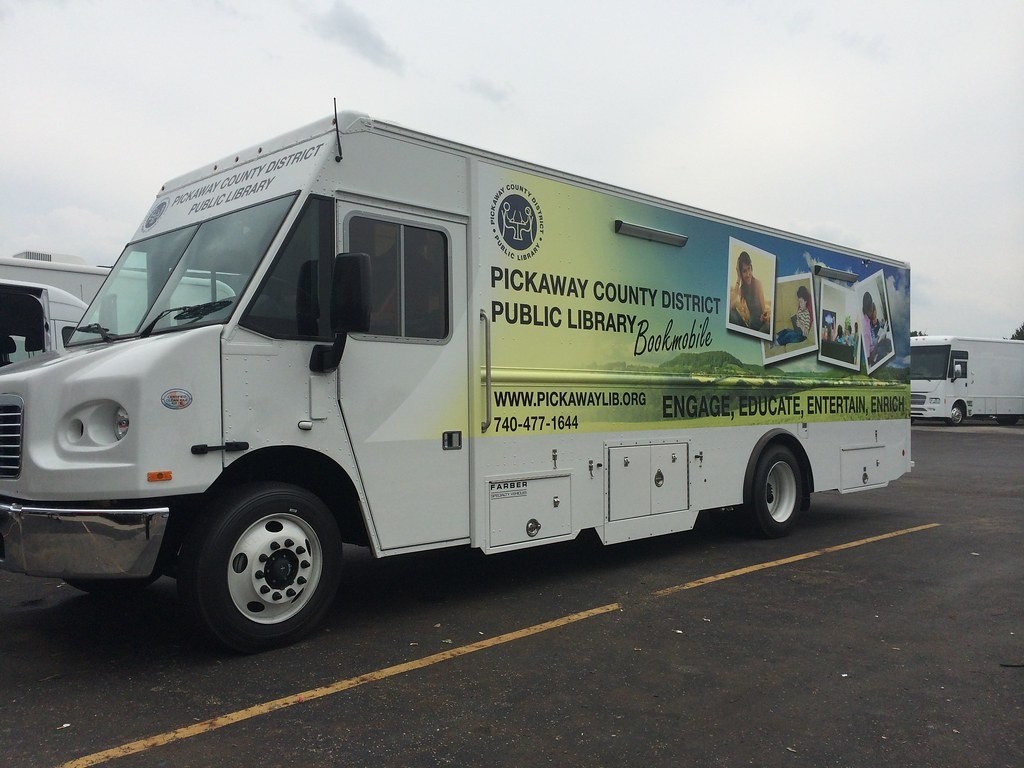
[[730, 251, 768, 330], [861, 291, 881, 368], [870, 302, 886, 345], [768, 290, 811, 350], [799, 286, 813, 323], [834, 323, 859, 362]]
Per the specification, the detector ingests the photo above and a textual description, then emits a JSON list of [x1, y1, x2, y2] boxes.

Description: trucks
[[909, 335, 1024, 428], [0, 252, 237, 344], [0, 94, 913, 647], [0, 278, 96, 371]]
